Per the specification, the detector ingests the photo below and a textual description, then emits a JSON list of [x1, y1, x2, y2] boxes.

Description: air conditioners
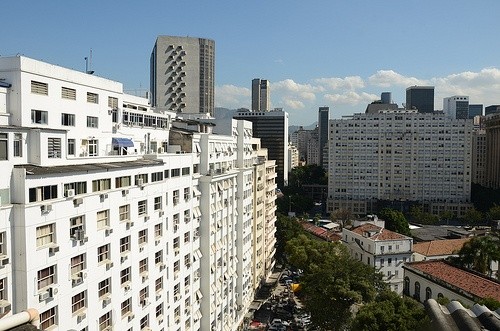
[[39, 183, 201, 331]]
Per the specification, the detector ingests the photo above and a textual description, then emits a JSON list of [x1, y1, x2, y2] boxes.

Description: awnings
[[112, 137, 135, 148]]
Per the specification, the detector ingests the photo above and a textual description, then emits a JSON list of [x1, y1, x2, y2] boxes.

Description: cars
[[245, 274, 313, 331]]
